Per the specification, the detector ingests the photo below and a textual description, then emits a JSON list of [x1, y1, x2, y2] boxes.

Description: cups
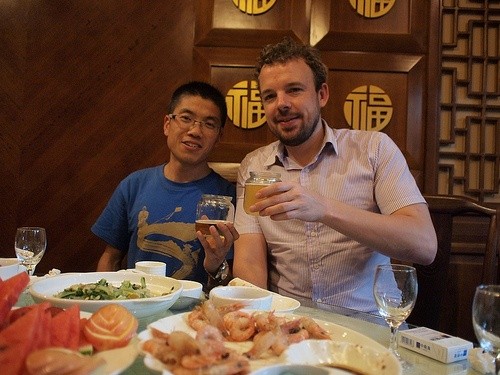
[[135, 260, 166, 276], [248, 362, 329, 375], [0, 258, 19, 281]]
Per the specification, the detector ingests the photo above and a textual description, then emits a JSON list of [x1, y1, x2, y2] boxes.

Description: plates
[[142, 313, 401, 375]]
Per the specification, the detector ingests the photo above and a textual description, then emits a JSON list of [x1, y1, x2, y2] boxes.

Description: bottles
[[194, 193, 235, 238], [242, 171, 283, 217]]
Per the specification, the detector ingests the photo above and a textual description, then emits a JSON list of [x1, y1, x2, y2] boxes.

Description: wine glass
[[472, 284, 500, 375], [14, 228, 46, 277], [373, 264, 418, 370]]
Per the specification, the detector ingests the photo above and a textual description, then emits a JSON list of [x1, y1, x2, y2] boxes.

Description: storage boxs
[[397, 327, 473, 365]]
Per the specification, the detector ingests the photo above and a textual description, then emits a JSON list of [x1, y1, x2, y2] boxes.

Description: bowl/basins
[[208, 286, 273, 317], [229, 278, 300, 314], [28, 271, 183, 319], [170, 280, 203, 310]]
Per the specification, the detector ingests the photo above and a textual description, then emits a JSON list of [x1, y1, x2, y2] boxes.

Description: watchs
[[205, 258, 229, 284]]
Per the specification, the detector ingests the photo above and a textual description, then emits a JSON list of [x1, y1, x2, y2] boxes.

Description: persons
[[91, 81, 240, 289], [233, 38, 438, 332]]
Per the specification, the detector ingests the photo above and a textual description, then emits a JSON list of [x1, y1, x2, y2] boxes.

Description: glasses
[[168, 112, 221, 131]]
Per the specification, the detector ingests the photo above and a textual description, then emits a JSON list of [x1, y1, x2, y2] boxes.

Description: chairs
[[392, 195, 500, 344]]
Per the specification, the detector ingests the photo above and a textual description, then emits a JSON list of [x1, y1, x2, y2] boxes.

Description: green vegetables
[[57, 275, 148, 300]]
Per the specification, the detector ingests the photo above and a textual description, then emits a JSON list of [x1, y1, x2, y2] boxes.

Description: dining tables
[[12, 275, 496, 375]]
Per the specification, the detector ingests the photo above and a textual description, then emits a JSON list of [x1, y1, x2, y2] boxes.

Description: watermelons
[[0, 271, 94, 375]]
